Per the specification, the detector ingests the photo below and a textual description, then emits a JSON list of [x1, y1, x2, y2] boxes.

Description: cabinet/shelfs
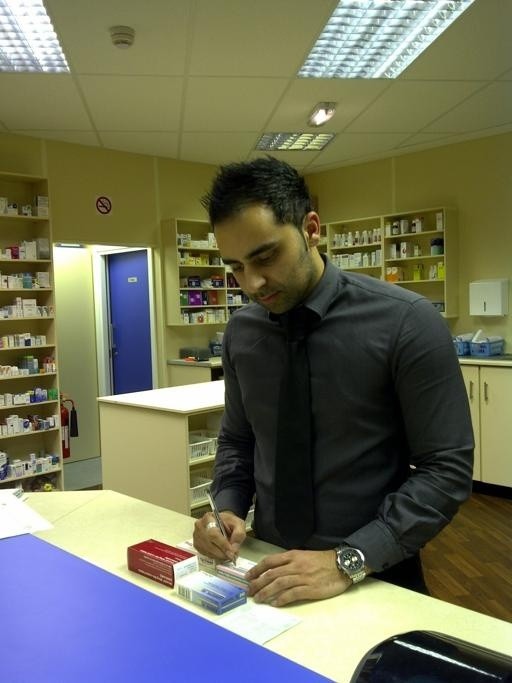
[[0, 170, 65, 491], [458, 354, 512, 500], [95, 379, 226, 517], [160, 205, 460, 327]]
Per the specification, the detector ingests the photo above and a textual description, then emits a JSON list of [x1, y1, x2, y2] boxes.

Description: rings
[[206, 522, 216, 530]]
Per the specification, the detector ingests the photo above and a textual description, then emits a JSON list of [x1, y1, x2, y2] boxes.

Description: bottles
[[47, 415, 55, 427], [24, 271, 33, 288], [181, 269, 187, 287], [392, 222, 399, 234], [332, 227, 381, 247], [411, 221, 415, 231]]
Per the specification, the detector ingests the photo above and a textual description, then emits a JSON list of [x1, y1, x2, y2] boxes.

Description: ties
[[274, 320, 316, 550]]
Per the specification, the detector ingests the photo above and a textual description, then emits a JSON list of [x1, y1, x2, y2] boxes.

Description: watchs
[[334, 545, 366, 584]]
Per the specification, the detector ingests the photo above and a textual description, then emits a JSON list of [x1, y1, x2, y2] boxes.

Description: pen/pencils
[[204, 489, 236, 566]]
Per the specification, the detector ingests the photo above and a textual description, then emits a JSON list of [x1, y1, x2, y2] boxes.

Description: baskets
[[451, 331, 504, 358]]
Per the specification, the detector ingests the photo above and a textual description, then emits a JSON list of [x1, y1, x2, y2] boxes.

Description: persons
[[193, 153, 473, 606]]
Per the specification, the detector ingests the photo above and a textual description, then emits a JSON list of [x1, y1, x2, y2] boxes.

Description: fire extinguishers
[[60, 391, 78, 459]]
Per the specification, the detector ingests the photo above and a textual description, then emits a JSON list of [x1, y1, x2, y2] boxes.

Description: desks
[[167, 356, 222, 387], [0, 489, 512, 683]]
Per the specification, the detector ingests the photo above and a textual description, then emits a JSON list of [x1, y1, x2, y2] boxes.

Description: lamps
[[307, 102, 337, 128]]
